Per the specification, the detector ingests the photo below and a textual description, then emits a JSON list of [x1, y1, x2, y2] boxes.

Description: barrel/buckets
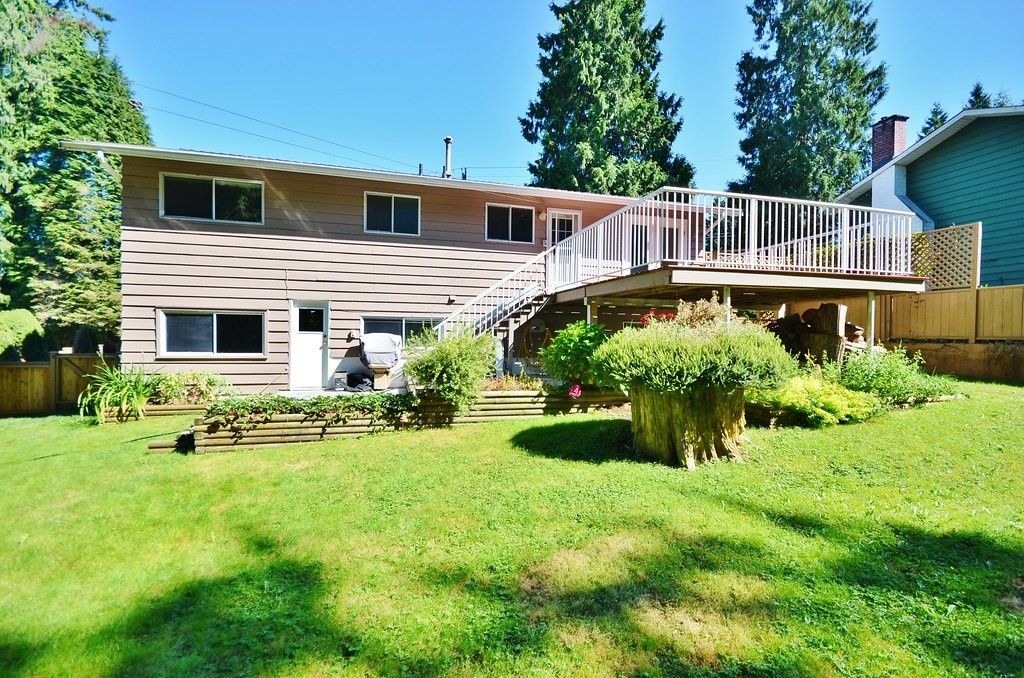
[[334, 373, 348, 391]]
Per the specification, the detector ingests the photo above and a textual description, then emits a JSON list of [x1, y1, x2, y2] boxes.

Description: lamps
[[539, 211, 547, 221]]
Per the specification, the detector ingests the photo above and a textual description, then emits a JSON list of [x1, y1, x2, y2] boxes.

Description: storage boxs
[[334, 373, 348, 390]]
